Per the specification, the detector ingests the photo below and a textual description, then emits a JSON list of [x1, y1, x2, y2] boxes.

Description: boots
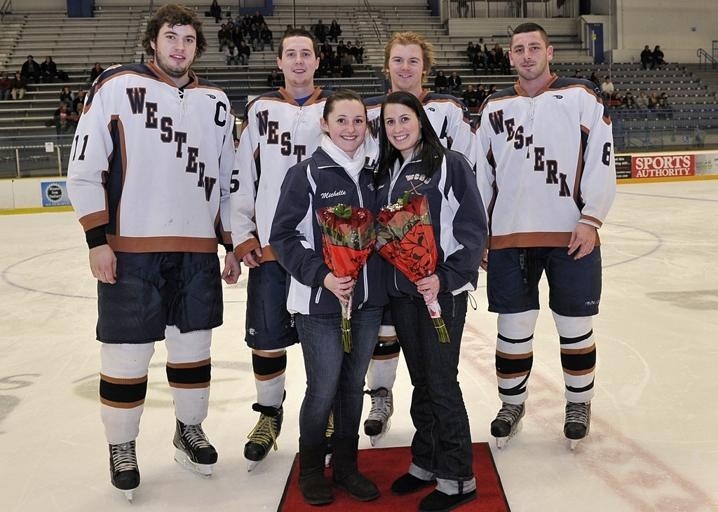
[[299, 438, 332, 505], [331, 434, 380, 501]]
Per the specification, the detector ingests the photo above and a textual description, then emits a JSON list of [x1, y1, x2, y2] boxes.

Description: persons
[[207, 0, 275, 66], [229, 28, 377, 472], [366, 32, 479, 446], [592, 71, 672, 122], [639, 44, 671, 74], [474, 23, 615, 451], [270, 12, 366, 84], [66, 5, 237, 502], [58, 61, 104, 130], [0, 56, 66, 103], [434, 31, 512, 113], [377, 93, 488, 511], [268, 90, 381, 505]]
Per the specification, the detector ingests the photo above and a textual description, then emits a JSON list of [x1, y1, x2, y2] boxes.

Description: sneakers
[[173, 417, 217, 464], [324, 407, 334, 454], [364, 387, 393, 435], [419, 490, 477, 512], [491, 403, 525, 437], [244, 390, 286, 461], [108, 440, 140, 490], [391, 472, 437, 495], [564, 400, 590, 439]]
